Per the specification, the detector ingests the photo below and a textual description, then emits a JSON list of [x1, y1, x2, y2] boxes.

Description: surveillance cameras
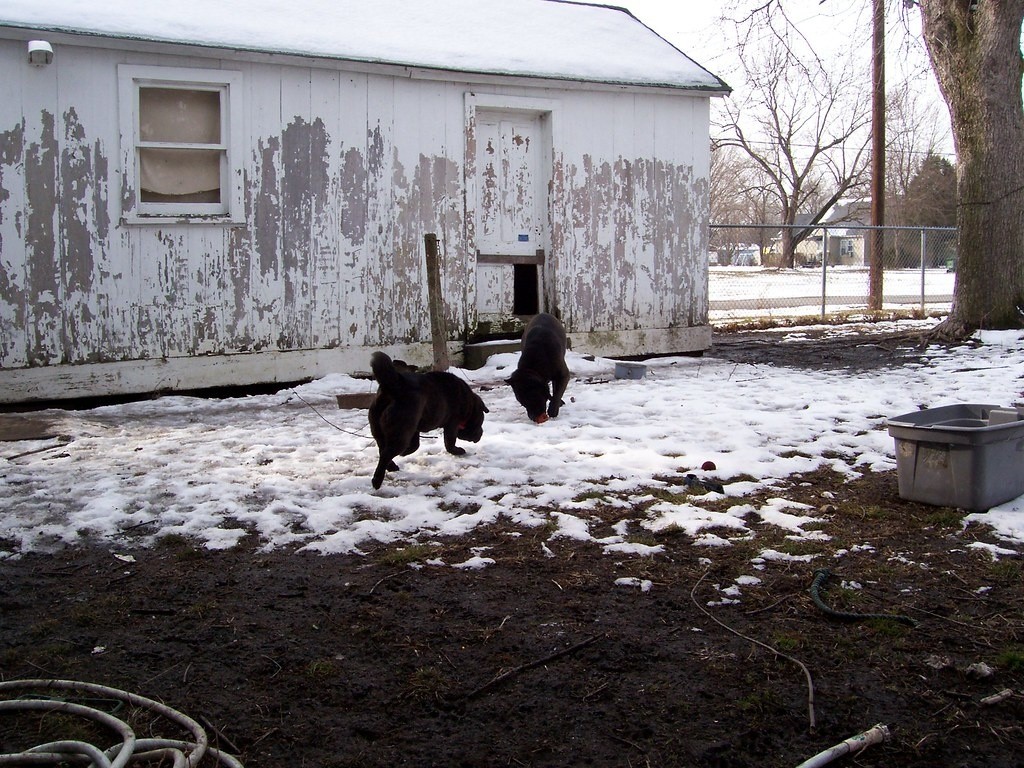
[[28, 40, 53, 66]]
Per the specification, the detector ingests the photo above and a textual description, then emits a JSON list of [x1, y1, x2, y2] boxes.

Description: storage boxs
[[888, 404, 1024, 513]]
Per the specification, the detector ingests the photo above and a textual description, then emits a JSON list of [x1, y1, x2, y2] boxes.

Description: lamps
[[28, 40, 54, 69]]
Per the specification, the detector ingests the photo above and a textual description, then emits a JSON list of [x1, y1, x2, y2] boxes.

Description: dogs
[[503, 313, 570, 423], [368, 351, 490, 490]]
